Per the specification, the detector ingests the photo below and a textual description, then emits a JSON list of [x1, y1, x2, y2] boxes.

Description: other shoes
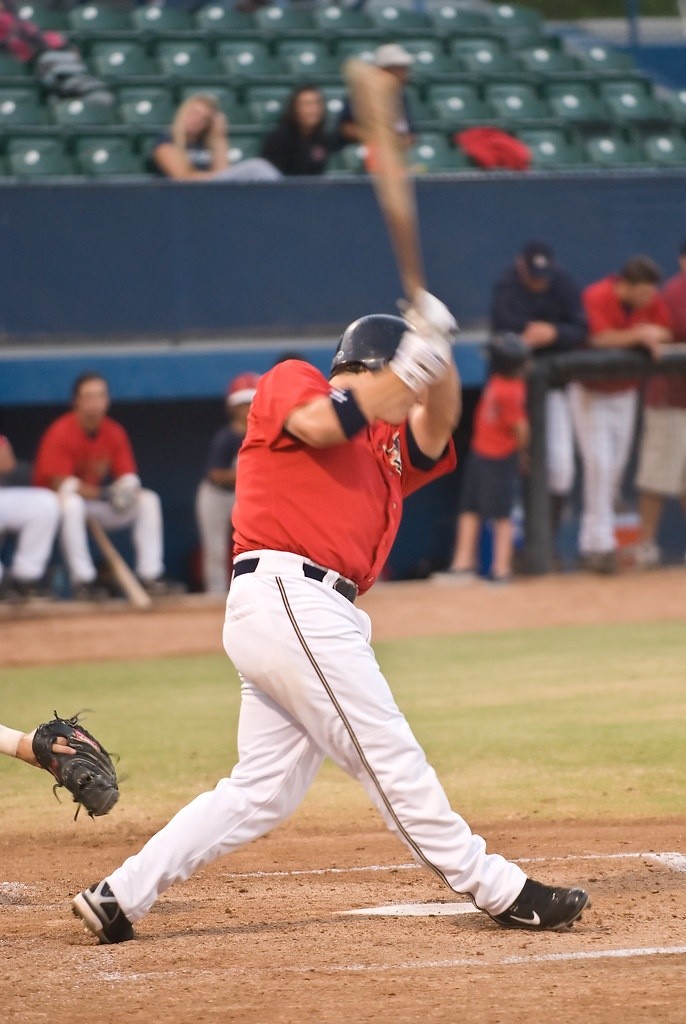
[[73, 584, 109, 603], [430, 567, 479, 580], [143, 575, 190, 597], [5, 573, 55, 604]]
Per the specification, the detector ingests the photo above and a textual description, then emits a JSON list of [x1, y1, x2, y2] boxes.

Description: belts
[[231, 561, 358, 602]]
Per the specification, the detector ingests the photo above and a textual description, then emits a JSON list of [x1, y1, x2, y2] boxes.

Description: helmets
[[331, 314, 414, 373]]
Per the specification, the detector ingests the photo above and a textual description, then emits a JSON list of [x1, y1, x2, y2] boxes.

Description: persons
[[567, 257, 673, 576], [622, 240, 686, 565], [0, 436, 60, 601], [196, 373, 262, 594], [491, 243, 590, 528], [336, 42, 415, 150], [146, 93, 228, 180], [0, 719, 120, 817], [267, 83, 331, 176], [431, 330, 532, 586], [73, 290, 592, 943], [34, 371, 169, 600]]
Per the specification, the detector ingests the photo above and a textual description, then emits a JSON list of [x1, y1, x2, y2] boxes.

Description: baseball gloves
[[31, 717, 120, 817]]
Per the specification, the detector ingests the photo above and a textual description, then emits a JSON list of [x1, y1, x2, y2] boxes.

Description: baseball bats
[[344, 54, 421, 297], [89, 516, 152, 607]]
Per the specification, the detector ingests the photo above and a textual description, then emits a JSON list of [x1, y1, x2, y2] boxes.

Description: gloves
[[389, 284, 459, 396]]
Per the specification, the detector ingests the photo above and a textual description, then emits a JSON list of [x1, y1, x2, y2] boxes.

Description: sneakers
[[73, 879, 134, 945], [489, 879, 589, 932]]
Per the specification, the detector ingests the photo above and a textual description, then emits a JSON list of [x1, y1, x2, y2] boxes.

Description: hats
[[524, 240, 559, 280], [379, 45, 413, 68], [227, 373, 261, 404]]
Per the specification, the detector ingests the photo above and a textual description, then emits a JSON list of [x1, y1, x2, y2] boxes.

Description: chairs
[[0, 0, 686, 178]]
